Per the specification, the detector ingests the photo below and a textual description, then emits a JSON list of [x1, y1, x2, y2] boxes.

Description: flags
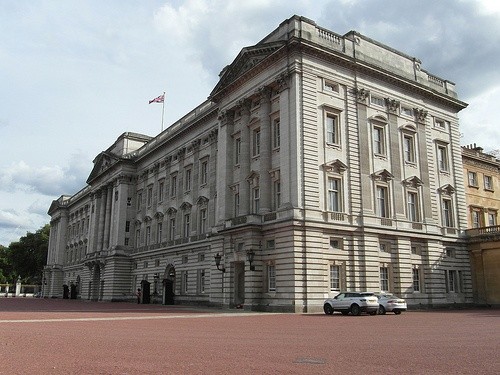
[[148, 93, 165, 105]]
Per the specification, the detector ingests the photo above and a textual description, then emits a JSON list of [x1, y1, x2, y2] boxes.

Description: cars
[[374, 291, 408, 315]]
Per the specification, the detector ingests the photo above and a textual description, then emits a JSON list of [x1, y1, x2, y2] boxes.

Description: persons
[[135, 288, 141, 304], [70, 281, 78, 299]]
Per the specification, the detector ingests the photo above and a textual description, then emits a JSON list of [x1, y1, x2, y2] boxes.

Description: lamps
[[247, 248, 255, 272], [215, 253, 226, 272]]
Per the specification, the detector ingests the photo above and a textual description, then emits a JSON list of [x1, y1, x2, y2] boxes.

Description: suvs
[[324, 293, 379, 316]]
